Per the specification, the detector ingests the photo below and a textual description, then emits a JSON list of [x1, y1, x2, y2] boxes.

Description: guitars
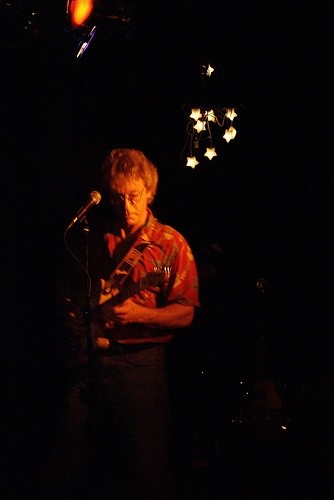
[[53, 265, 170, 365]]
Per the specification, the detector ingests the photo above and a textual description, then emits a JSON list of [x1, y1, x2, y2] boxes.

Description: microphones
[[66, 190, 102, 231]]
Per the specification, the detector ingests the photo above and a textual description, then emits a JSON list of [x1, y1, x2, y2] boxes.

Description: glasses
[[109, 189, 145, 205]]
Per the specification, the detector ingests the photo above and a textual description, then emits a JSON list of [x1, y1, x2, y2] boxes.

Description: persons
[[53, 146, 200, 500]]
